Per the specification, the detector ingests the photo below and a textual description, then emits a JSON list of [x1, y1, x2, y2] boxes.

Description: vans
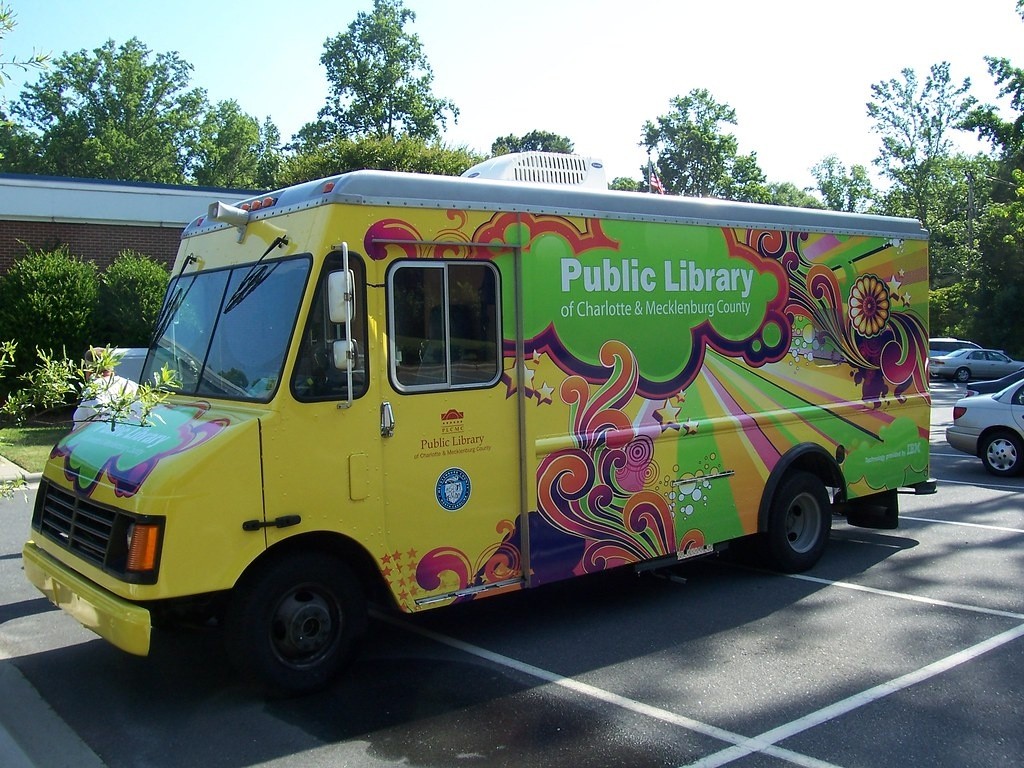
[[928, 336, 984, 378]]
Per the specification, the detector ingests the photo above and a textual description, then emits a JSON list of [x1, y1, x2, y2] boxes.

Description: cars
[[946, 365, 1024, 477], [929, 348, 1024, 383]]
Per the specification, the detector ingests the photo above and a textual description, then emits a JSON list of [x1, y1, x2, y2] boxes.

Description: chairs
[[413, 299, 479, 384]]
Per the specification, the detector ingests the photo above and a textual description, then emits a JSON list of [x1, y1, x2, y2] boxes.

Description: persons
[[73, 347, 139, 431]]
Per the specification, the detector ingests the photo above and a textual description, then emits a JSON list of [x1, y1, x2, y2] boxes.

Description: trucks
[[22, 152, 936, 708]]
